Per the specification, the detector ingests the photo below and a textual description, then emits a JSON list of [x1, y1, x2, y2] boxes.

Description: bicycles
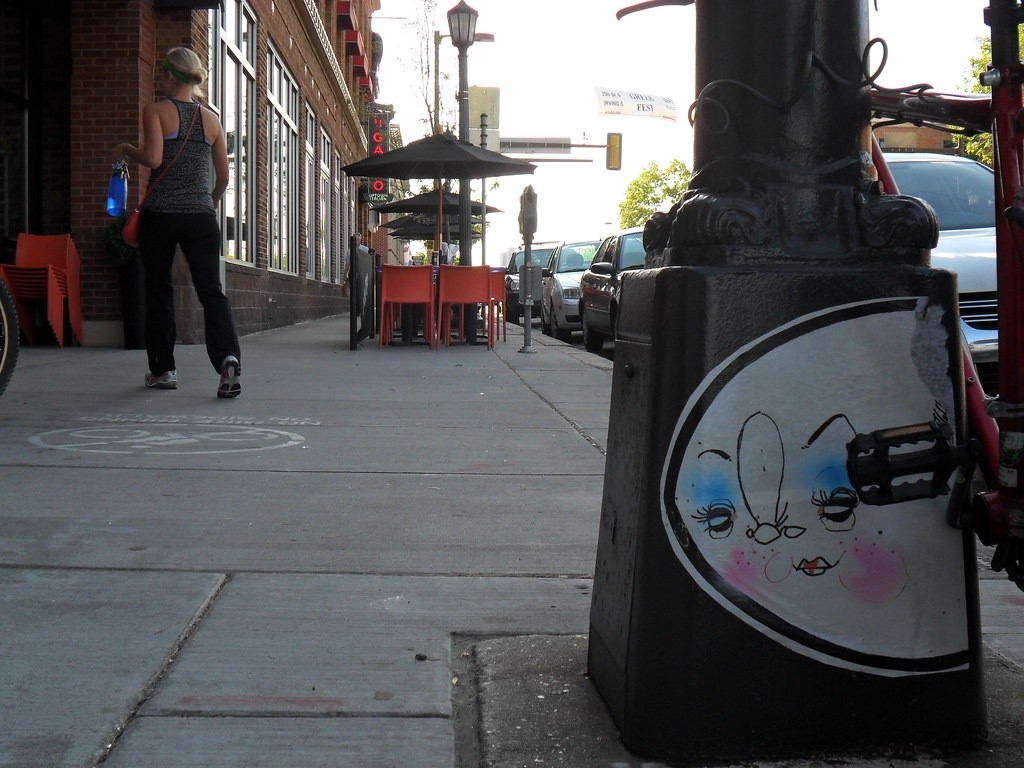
[[616, 0, 1024, 593], [0, 280, 20, 398]]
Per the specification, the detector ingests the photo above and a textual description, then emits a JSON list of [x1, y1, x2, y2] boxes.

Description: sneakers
[[217, 355, 241, 399], [145, 370, 178, 389]]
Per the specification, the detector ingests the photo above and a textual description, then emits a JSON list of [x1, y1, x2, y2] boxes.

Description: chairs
[[436, 264, 506, 351], [378, 264, 435, 350], [0, 233, 83, 349], [566, 253, 585, 269]]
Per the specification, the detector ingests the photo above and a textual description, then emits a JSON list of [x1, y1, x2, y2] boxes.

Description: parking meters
[[518, 185, 537, 354]]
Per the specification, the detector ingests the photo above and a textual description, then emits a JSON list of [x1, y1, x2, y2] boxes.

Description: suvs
[[505, 247, 554, 324]]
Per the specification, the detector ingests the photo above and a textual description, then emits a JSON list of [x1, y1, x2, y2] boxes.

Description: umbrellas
[[342, 134, 537, 281], [369, 189, 504, 251]]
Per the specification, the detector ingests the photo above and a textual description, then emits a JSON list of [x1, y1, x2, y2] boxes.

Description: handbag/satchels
[[120, 209, 141, 247]]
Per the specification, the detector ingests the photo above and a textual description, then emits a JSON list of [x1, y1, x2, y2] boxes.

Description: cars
[[872, 149, 1001, 430], [540, 241, 602, 342], [577, 226, 647, 353], [489, 266, 508, 273]]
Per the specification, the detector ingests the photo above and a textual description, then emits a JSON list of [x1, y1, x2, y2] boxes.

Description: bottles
[[107, 162, 128, 217]]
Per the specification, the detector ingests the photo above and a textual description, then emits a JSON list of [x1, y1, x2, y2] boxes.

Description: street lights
[[606, 221, 625, 232], [433, 29, 495, 349], [447, 0, 478, 346]]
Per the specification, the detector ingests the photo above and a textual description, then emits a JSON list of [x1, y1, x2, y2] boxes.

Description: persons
[[111, 47, 242, 398], [342, 233, 370, 316]]
